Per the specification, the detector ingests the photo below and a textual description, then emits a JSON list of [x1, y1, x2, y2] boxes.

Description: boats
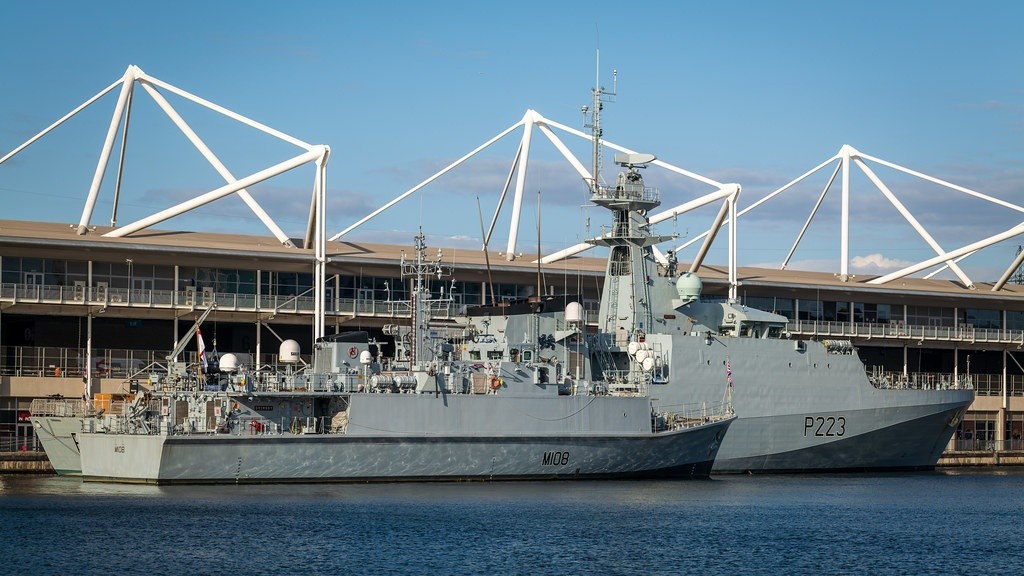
[[21, 45, 977, 487]]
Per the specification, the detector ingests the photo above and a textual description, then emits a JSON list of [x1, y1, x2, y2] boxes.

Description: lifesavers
[[490, 377, 500, 389]]
